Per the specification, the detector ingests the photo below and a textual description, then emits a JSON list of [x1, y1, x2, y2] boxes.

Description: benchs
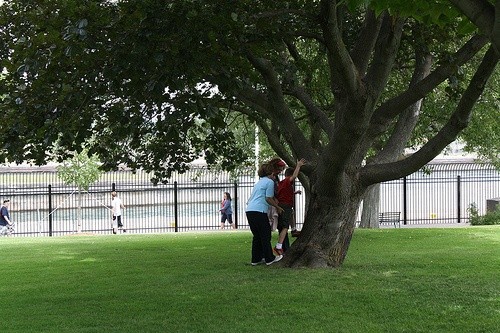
[[378, 212, 401, 228]]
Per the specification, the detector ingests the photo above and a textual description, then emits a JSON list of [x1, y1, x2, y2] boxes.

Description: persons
[[217, 192, 236, 230], [0, 200, 15, 237], [268, 158, 307, 258], [246, 163, 283, 266], [111, 192, 127, 235]]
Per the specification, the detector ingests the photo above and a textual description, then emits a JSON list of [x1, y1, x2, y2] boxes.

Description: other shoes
[[273, 248, 286, 257], [266, 255, 283, 265], [251, 258, 266, 266]]
